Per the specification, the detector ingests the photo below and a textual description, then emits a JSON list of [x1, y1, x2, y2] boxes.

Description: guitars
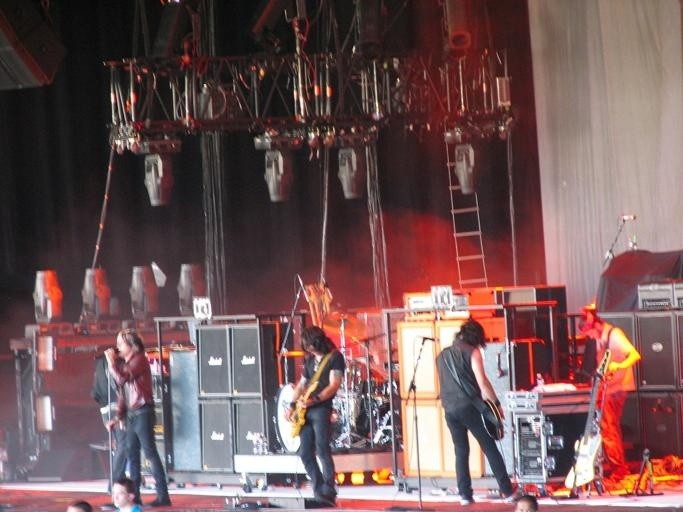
[[290, 381, 318, 437], [565, 349, 611, 489], [481, 398, 504, 439]]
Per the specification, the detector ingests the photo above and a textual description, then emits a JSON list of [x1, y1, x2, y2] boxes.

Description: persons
[[112, 479, 143, 512], [578, 311, 641, 483], [435, 320, 518, 505], [101, 329, 172, 510], [515, 495, 538, 512], [284, 325, 345, 507], [90, 352, 128, 493], [67, 501, 92, 512]]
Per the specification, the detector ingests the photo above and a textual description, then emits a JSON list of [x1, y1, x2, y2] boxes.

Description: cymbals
[[324, 312, 363, 333]]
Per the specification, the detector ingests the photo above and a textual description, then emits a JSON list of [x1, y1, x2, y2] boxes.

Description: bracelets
[[289, 401, 297, 411], [313, 393, 322, 404], [494, 400, 500, 407]]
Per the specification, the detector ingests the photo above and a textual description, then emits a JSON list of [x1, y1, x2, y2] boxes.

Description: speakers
[[228, 324, 294, 397], [637, 393, 679, 460], [202, 403, 232, 473], [594, 313, 635, 394], [632, 312, 678, 393], [232, 401, 288, 456], [198, 326, 233, 398], [672, 312, 683, 393], [618, 391, 642, 460], [268, 497, 334, 509], [166, 350, 202, 473]]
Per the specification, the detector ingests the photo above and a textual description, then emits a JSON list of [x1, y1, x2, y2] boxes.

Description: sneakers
[[503, 482, 520, 502], [459, 496, 476, 506], [146, 498, 172, 507]]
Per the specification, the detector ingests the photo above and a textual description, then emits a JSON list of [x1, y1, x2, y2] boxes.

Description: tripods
[[331, 320, 374, 449]]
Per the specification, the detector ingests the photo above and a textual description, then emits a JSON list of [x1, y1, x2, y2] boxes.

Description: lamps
[[337, 146, 365, 201], [261, 148, 292, 205], [453, 143, 480, 195], [143, 152, 171, 208]]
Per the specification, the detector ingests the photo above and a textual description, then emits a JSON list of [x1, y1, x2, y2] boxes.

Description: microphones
[[622, 214, 636, 220], [96, 348, 119, 359], [423, 337, 440, 344]]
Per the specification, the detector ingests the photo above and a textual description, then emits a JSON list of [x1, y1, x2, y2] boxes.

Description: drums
[[273, 383, 301, 454]]
